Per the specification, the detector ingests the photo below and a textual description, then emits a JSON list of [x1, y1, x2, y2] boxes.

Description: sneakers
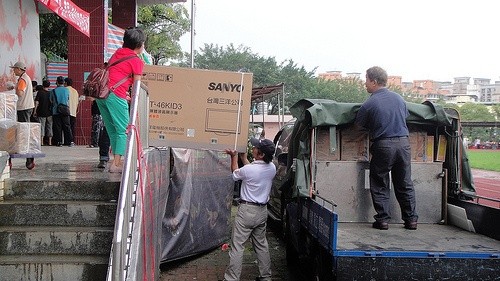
[[98, 160, 106, 168]]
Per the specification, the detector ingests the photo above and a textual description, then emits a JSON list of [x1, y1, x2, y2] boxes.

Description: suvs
[[268, 122, 293, 241]]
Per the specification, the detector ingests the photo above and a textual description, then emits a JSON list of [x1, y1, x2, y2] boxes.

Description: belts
[[379, 136, 408, 139], [241, 199, 267, 206]]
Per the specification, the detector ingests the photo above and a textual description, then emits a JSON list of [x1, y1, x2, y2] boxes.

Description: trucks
[[287, 102, 500, 281]]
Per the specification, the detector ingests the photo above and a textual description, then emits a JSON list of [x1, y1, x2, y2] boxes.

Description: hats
[[11, 63, 26, 70], [251, 139, 275, 156]]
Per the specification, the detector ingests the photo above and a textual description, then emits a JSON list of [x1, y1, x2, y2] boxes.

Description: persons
[[96, 29, 146, 172], [356, 66, 419, 229], [224, 138, 277, 281], [7, 60, 111, 169], [463, 135, 500, 150]]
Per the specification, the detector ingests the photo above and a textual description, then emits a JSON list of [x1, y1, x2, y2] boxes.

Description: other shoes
[[42, 142, 76, 148], [218, 279, 223, 281], [86, 144, 98, 148], [254, 277, 263, 281], [109, 165, 123, 172]]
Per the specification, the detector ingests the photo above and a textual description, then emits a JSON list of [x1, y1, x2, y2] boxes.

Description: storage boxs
[[130, 64, 253, 154], [0, 93, 41, 202]]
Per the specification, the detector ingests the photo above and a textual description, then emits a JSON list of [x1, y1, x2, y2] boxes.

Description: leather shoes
[[405, 221, 417, 229], [373, 221, 388, 230]]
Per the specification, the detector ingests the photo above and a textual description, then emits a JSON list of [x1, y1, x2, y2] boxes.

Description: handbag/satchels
[[56, 104, 69, 115]]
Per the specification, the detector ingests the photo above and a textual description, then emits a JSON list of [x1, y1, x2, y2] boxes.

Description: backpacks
[[83, 56, 140, 99]]
